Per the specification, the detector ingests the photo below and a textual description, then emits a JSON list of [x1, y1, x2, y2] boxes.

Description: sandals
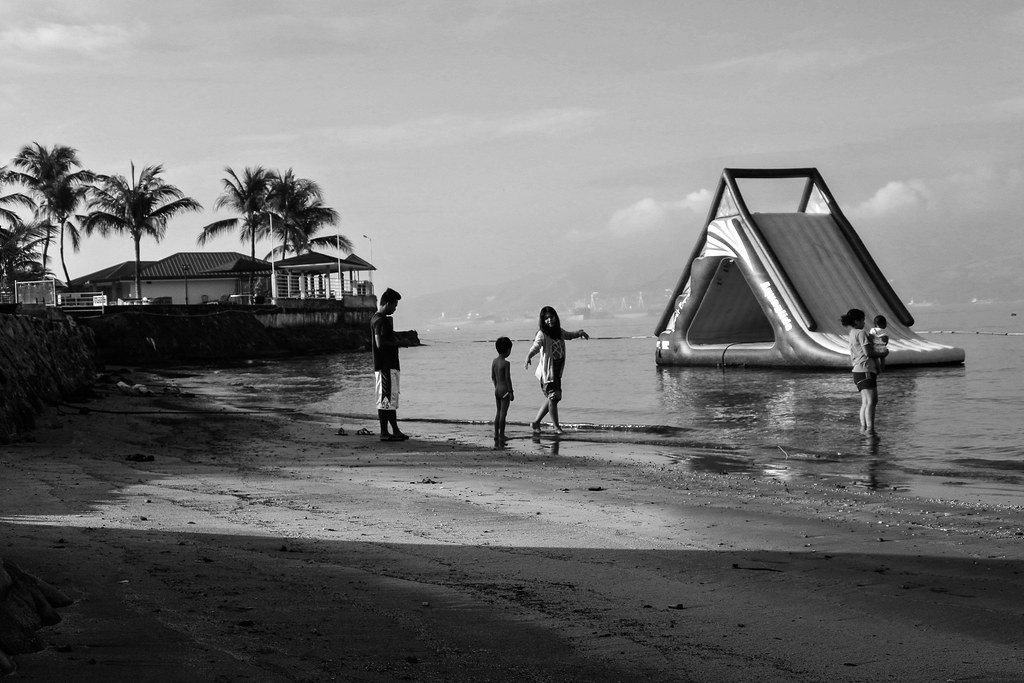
[[124, 454, 155, 462]]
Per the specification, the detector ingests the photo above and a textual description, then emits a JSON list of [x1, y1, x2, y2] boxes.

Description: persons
[[492, 336, 514, 441], [524, 305, 591, 435], [841, 308, 890, 437], [370, 287, 416, 442], [867, 314, 889, 374]]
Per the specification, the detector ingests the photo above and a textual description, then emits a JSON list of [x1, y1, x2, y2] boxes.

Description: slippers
[[334, 428, 348, 436], [354, 428, 375, 435], [380, 433, 409, 441]]
